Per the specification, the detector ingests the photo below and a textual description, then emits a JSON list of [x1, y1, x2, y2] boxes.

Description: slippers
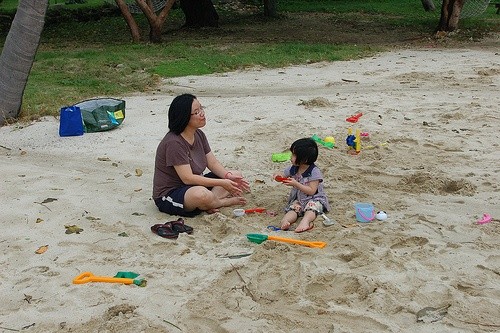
[[165, 217, 194, 234], [151, 222, 181, 240]]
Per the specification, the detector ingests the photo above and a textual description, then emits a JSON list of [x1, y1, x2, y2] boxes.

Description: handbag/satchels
[[73, 97, 125, 133], [58, 106, 84, 136]]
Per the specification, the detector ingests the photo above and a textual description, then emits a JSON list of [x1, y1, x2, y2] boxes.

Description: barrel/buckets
[[355, 202, 375, 223]]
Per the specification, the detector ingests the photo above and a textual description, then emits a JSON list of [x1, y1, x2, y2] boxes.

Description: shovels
[[321, 214, 335, 226], [245, 233, 326, 249]]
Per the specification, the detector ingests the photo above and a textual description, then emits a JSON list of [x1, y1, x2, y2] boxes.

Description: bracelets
[[225, 172, 233, 179]]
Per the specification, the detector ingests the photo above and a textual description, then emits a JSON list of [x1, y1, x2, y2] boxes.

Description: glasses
[[189, 106, 209, 118]]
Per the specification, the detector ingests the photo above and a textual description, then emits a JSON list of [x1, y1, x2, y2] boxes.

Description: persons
[[280, 138, 331, 232], [153, 94, 251, 218]]
